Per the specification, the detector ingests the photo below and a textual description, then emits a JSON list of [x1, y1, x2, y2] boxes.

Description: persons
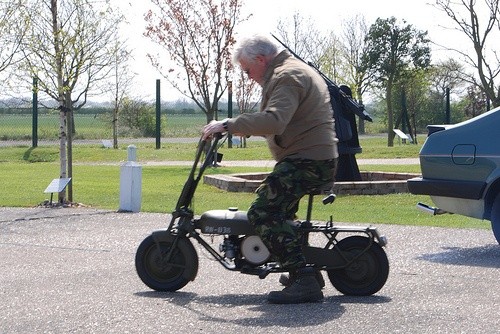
[[201, 36, 338, 305]]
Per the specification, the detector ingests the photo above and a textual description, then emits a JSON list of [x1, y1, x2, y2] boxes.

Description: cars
[[407, 106, 497, 240]]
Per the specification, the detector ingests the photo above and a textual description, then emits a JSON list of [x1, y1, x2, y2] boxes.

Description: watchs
[[222, 118, 230, 132]]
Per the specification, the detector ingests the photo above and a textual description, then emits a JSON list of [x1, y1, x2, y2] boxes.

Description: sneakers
[[268, 272, 325, 302], [279, 271, 325, 287]]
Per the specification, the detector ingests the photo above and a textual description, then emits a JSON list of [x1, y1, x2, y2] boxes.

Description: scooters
[[135, 121, 390, 295]]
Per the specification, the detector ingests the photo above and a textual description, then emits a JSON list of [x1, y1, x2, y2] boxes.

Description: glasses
[[245, 62, 253, 74]]
